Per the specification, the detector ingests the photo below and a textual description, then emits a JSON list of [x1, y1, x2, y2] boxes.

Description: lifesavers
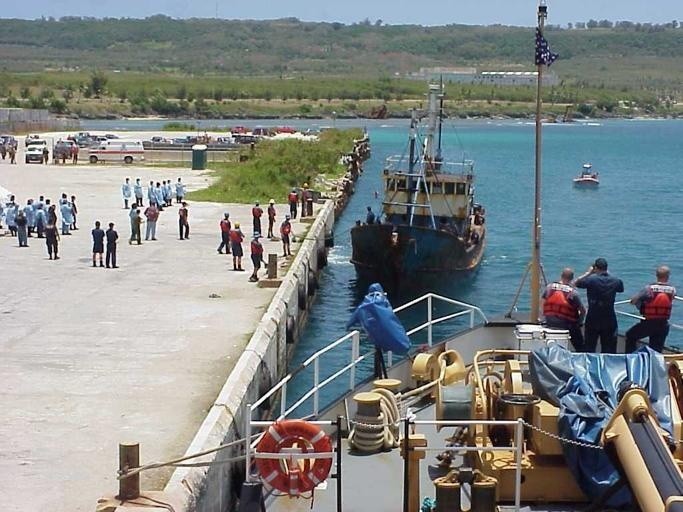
[[255, 419, 333, 499]]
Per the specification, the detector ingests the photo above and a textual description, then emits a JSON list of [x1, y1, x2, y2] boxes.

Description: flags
[[535, 27, 559, 66]]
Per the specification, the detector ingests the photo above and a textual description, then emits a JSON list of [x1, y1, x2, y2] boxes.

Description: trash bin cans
[[192, 144, 207, 170]]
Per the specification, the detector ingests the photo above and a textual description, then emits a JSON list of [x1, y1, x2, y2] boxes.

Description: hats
[[268, 199, 275, 204], [253, 231, 261, 238], [234, 221, 240, 228]]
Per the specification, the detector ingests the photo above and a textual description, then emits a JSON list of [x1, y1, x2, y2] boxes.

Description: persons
[[366, 206, 375, 224], [217, 182, 309, 282], [3, 177, 190, 268], [0, 134, 80, 165], [571, 258, 624, 354], [541, 268, 585, 352], [625, 266, 677, 353]]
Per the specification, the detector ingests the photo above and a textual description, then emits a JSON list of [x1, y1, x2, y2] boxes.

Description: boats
[[347, 76, 487, 286], [571, 162, 599, 188], [240, 2, 682, 512]]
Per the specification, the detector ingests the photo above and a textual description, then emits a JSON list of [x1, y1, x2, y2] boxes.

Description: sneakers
[[268, 235, 274, 238], [54, 257, 60, 260], [10, 227, 78, 247], [249, 275, 258, 282], [179, 236, 190, 240], [49, 258, 54, 259], [128, 240, 142, 245], [217, 248, 232, 254], [144, 238, 157, 241], [234, 267, 245, 271], [92, 262, 119, 268], [124, 201, 182, 210], [284, 253, 291, 256]]
